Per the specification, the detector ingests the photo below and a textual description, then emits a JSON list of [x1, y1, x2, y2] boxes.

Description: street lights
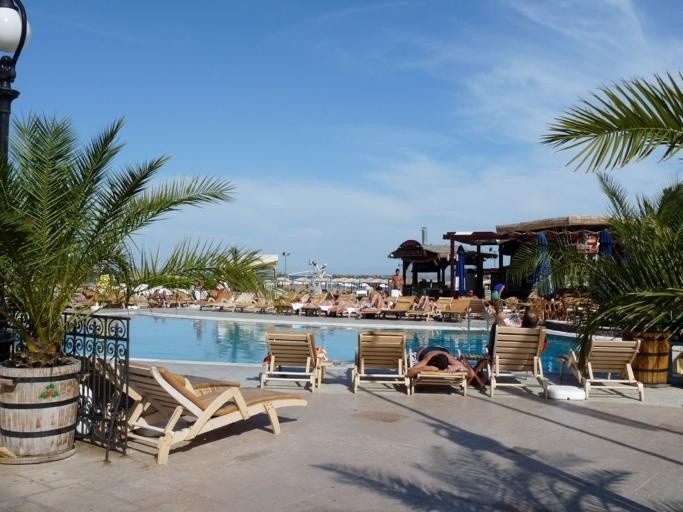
[[282, 251, 290, 290], [0, 1, 32, 364]]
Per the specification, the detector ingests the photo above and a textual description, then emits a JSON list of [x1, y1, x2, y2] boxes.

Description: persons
[[205, 283, 224, 303], [414, 294, 438, 313], [368, 286, 382, 309], [490, 283, 507, 306], [485, 300, 510, 362], [405, 343, 469, 377], [388, 284, 405, 298], [466, 289, 479, 300], [519, 311, 548, 356], [358, 290, 390, 310], [389, 269, 404, 289]]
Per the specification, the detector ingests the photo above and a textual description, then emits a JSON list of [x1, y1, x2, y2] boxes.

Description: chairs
[[199, 291, 487, 323]]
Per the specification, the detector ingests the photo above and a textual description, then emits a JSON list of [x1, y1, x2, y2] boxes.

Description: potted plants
[[0, 106, 269, 481]]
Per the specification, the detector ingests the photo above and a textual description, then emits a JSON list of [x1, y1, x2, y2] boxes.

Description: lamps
[[0, 1, 37, 102]]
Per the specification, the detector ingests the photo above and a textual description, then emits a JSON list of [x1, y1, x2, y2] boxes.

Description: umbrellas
[[530, 231, 554, 299], [452, 243, 467, 298], [593, 227, 618, 312], [273, 276, 392, 294]]
